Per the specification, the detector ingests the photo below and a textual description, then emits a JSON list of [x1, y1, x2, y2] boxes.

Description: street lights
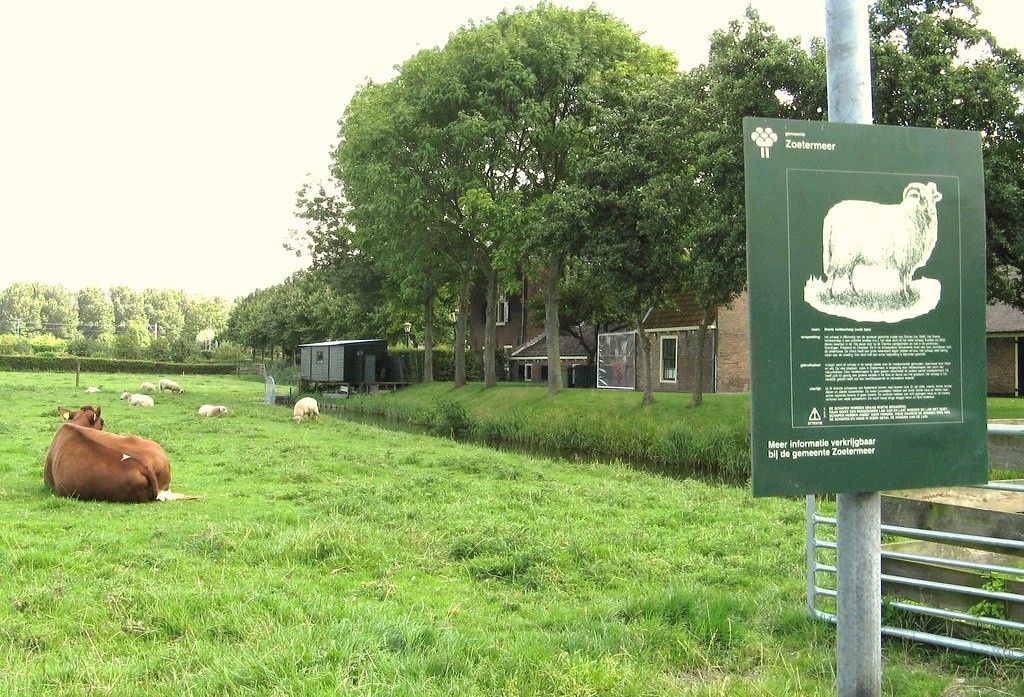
[[404, 322, 412, 349]]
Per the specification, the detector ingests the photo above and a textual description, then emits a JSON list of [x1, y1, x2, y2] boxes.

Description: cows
[[43, 405, 171, 503]]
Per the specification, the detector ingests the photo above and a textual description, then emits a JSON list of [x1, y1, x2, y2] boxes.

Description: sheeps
[[823, 182, 942, 304], [120, 391, 154, 406], [198, 404, 227, 417], [292, 397, 320, 423], [85, 386, 100, 393], [159, 379, 183, 394], [140, 382, 156, 392]]
